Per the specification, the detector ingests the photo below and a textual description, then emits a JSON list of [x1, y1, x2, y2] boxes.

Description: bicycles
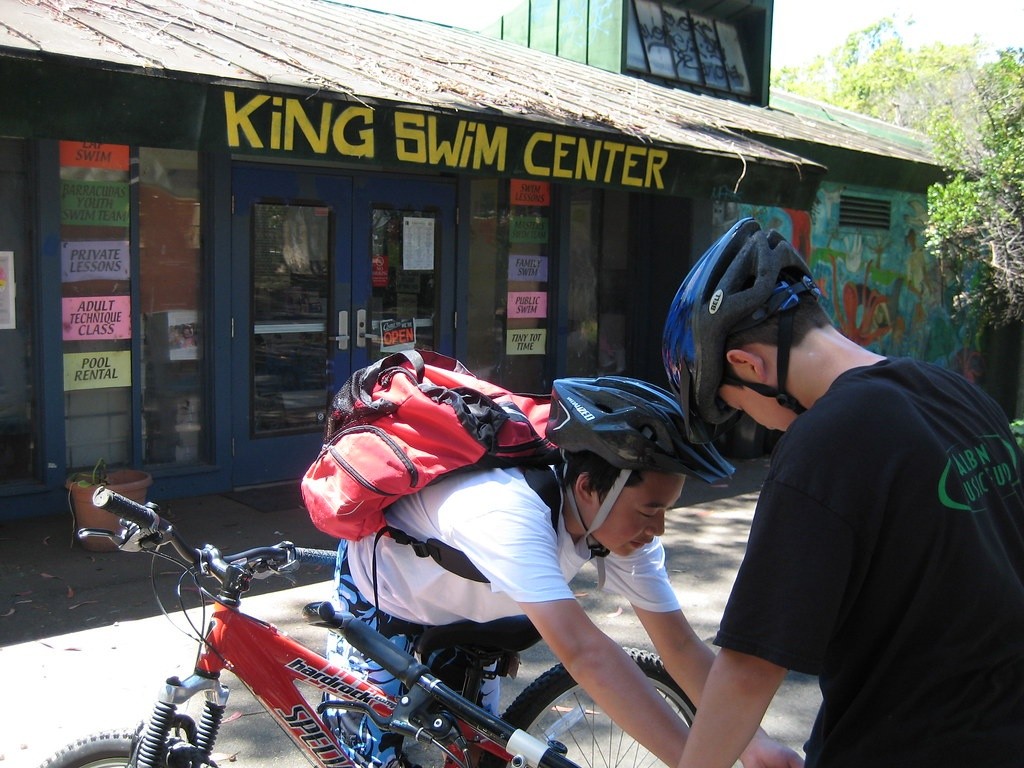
[[40, 485, 697, 768]]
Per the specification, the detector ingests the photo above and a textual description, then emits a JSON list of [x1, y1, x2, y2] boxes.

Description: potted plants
[[66, 459, 155, 552]]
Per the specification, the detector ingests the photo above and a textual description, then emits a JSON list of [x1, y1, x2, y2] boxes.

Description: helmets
[[661, 216, 808, 445], [544, 375, 736, 485]]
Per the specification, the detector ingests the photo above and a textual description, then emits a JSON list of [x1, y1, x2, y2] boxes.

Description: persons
[[333, 376, 808, 768], [660, 219, 1024, 768]]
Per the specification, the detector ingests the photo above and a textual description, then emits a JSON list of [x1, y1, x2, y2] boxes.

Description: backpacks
[[301, 349, 561, 584]]
